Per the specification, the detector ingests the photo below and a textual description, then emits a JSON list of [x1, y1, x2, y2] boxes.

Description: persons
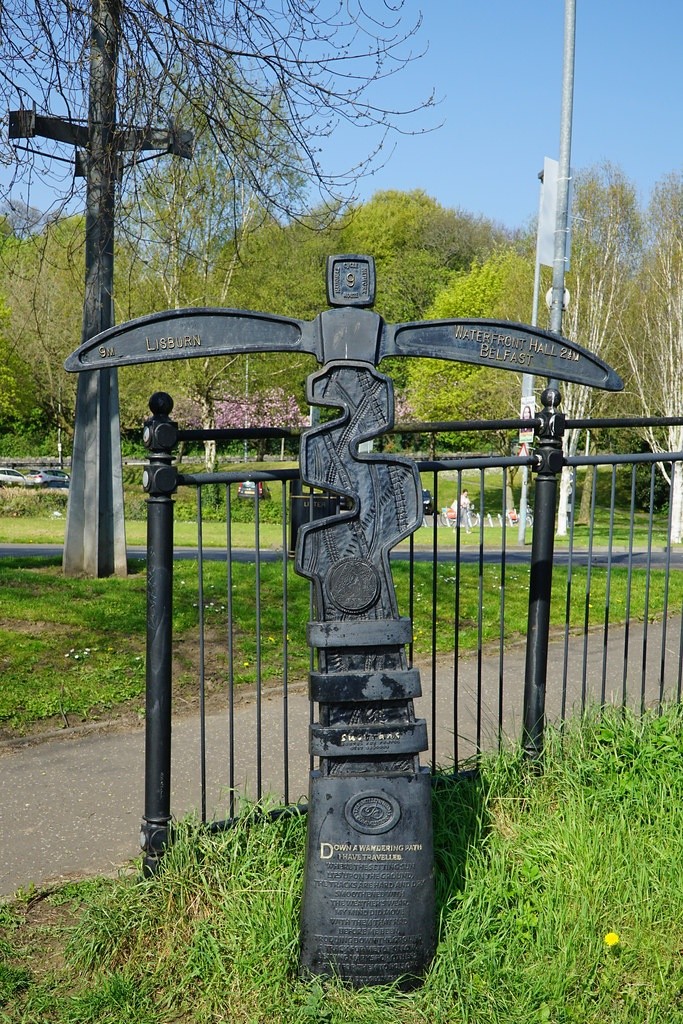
[[520, 405, 533, 432], [452, 488, 472, 535]]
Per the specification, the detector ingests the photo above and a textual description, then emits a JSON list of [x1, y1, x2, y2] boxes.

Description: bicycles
[[440, 504, 479, 527]]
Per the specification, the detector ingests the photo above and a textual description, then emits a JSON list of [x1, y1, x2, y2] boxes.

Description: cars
[[0, 467, 33, 488], [238, 481, 271, 501], [422, 489, 434, 516]]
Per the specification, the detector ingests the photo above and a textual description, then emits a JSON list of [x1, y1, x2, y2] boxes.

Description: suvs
[[26, 468, 70, 488]]
